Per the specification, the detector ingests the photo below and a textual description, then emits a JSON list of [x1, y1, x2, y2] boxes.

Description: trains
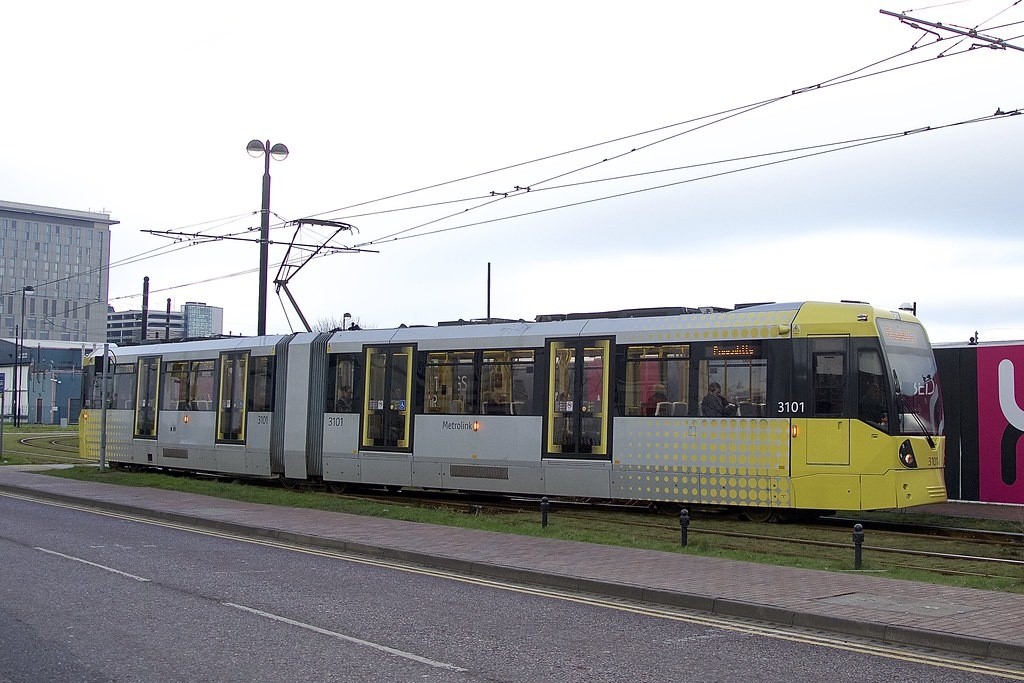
[[76, 299, 950, 526]]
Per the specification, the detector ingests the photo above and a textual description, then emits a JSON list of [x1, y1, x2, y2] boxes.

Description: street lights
[[245, 138, 289, 337], [17, 286, 35, 429]]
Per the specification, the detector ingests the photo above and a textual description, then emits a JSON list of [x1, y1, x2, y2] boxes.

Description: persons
[[393, 388, 402, 399], [335, 386, 353, 412], [556, 384, 729, 418]]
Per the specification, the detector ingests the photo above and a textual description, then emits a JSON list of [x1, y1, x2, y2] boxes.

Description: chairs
[[655, 402, 766, 418], [481, 401, 528, 416], [368, 412, 406, 447], [175, 399, 213, 411], [125, 400, 131, 409], [554, 410, 605, 454]]
